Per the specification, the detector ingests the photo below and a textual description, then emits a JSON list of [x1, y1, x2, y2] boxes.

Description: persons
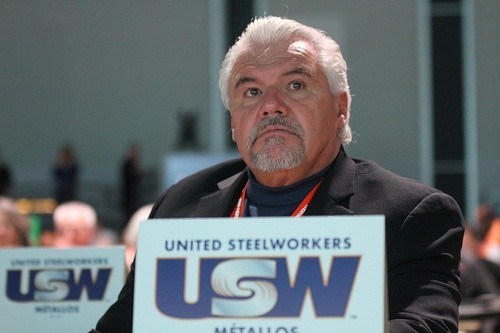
[[458, 203, 499, 333], [87, 14, 467, 333], [41, 143, 158, 275], [0, 166, 31, 248]]
